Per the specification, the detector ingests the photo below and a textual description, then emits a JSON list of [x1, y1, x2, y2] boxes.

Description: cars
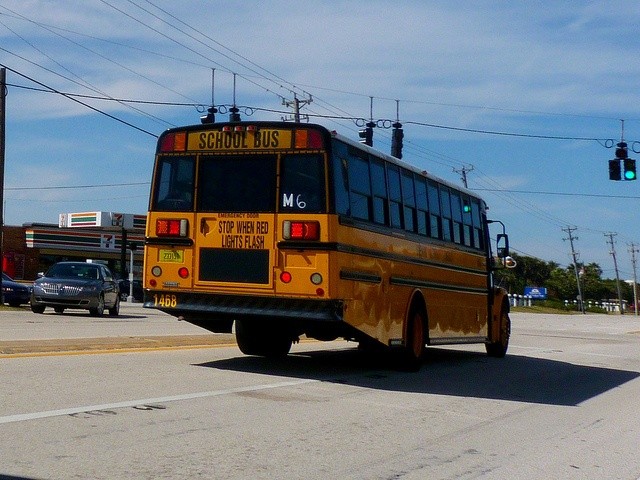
[[1, 273, 32, 306], [30, 261, 120, 316], [117, 279, 143, 301]]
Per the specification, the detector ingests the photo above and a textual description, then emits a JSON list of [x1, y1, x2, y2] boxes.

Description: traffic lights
[[359, 129, 372, 147], [200, 114, 215, 125], [624, 159, 636, 180]]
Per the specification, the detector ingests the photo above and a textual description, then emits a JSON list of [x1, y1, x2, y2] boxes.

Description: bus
[[143, 121, 510, 371]]
